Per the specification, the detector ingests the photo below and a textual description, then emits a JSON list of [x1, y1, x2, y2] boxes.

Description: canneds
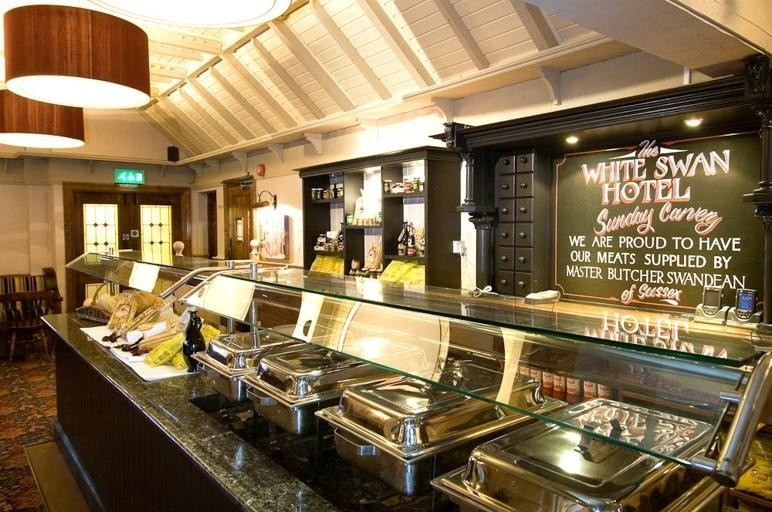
[[737, 291, 753, 312]]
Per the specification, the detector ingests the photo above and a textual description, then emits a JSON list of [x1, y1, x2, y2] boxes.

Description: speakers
[[167, 146, 179, 162]]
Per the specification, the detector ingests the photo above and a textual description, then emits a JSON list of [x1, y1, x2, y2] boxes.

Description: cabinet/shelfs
[[292, 145, 463, 288]]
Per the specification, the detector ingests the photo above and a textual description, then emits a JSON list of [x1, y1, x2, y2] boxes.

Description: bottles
[[181, 308, 206, 371], [394, 220, 418, 259]]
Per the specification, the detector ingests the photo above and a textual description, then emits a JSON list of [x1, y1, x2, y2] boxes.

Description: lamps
[[250, 190, 277, 209], [3, 4, 151, 109], [0, 88, 86, 149]]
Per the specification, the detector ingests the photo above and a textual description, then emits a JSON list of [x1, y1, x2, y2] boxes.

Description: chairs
[[0, 266, 63, 361]]
